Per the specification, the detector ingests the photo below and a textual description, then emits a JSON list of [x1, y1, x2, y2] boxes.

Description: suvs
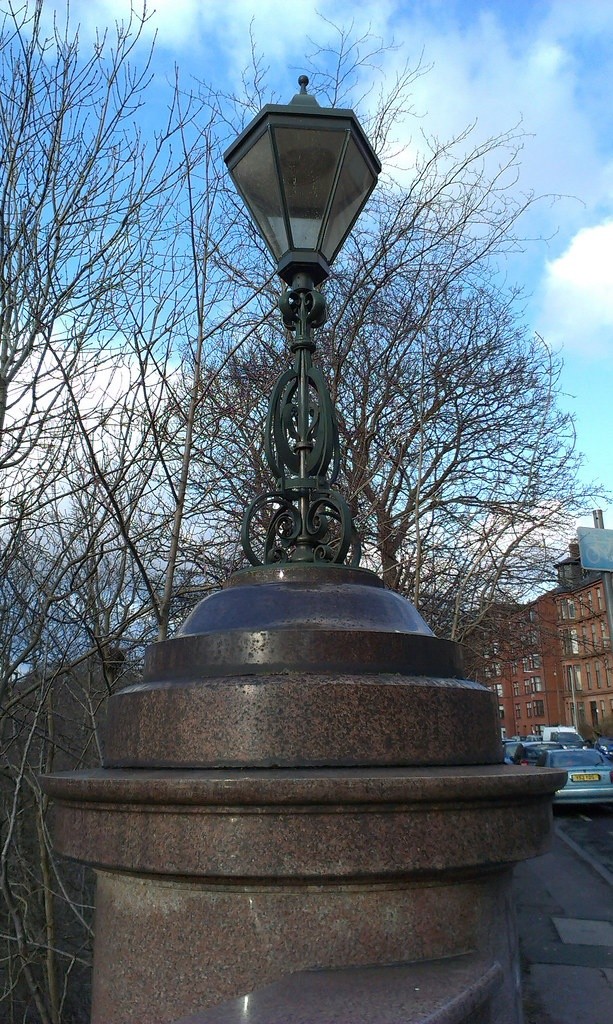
[[549, 730, 591, 750]]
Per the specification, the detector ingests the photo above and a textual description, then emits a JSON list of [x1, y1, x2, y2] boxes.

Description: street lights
[[223, 73, 385, 573]]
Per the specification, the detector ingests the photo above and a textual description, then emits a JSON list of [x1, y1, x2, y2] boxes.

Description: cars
[[503, 734, 566, 767], [594, 737, 613, 757], [537, 748, 613, 812]]
